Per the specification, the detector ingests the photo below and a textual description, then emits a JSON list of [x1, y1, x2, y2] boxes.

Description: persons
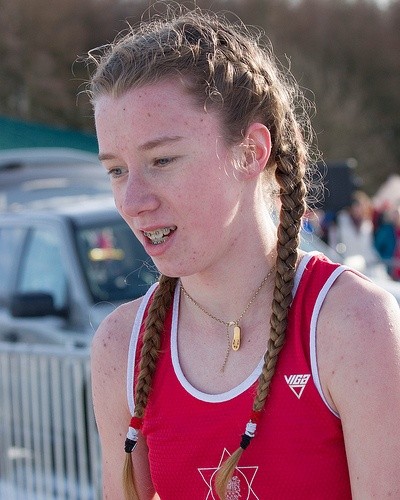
[[303, 161, 400, 298], [88, 10, 400, 500]]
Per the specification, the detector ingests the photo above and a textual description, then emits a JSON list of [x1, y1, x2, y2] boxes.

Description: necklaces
[[180, 266, 276, 373]]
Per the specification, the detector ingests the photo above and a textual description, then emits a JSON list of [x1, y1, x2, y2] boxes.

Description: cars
[[0, 196, 399, 480], [1, 148, 117, 210]]
